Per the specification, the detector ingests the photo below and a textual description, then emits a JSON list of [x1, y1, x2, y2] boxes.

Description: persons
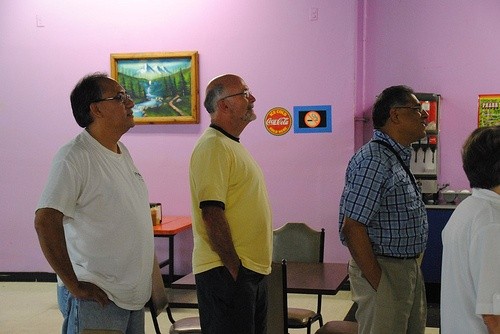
[[336, 84, 430, 334], [190, 73, 273, 334], [440, 123, 500, 334], [34, 74, 155, 334]]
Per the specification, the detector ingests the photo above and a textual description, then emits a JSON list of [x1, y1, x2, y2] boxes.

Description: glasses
[[219, 90, 252, 100], [389, 103, 422, 113], [91, 92, 130, 103]]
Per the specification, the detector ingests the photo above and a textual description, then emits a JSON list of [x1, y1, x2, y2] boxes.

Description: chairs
[[149, 222, 358, 334]]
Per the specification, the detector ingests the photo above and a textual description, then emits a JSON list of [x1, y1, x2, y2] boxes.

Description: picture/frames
[[110, 50, 201, 125]]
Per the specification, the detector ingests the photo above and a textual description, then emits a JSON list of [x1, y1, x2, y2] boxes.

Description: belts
[[376, 254, 420, 259]]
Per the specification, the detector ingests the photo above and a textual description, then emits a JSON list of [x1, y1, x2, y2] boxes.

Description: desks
[[420, 204, 456, 289], [153, 216, 192, 289], [172, 262, 349, 295]]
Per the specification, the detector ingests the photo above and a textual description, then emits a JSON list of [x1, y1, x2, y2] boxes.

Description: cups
[[150, 203, 163, 226]]
